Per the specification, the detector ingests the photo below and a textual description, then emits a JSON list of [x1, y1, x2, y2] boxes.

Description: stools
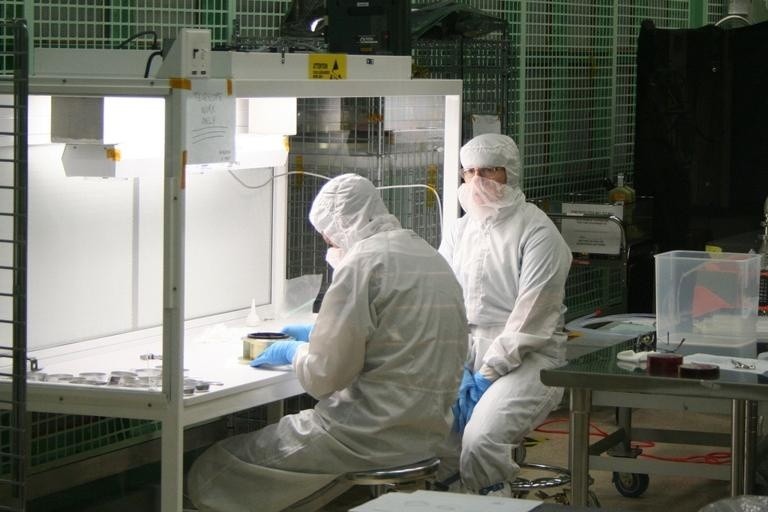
[[345, 458, 441, 497]]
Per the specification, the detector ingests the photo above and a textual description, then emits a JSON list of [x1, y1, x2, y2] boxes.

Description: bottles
[[607, 172, 637, 204]]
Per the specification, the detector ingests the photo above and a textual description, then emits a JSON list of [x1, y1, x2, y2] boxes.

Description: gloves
[[451, 366, 492, 432], [280, 326, 313, 341], [249, 340, 302, 367]]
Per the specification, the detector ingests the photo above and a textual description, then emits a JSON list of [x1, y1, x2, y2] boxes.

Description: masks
[[325, 248, 344, 269]]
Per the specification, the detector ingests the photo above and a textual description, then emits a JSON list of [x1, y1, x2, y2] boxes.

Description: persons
[[186, 172, 471, 511], [437, 132, 575, 496]]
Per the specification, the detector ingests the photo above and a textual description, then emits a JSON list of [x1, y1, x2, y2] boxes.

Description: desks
[[540, 331, 768, 510], [0, 320, 304, 511]]
